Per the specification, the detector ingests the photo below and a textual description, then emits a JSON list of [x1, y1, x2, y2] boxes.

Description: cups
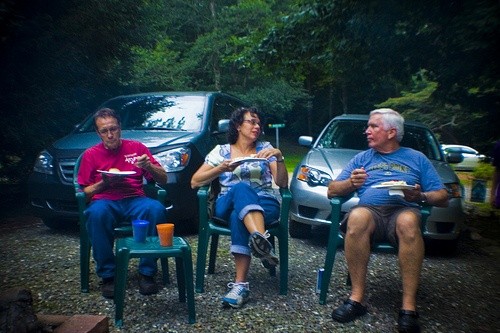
[[133, 220, 149, 242], [157, 224, 174, 246]]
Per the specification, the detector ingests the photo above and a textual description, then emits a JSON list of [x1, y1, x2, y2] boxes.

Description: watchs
[[277, 156, 284, 163]]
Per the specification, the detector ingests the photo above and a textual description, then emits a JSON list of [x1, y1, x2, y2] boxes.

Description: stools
[[114, 236, 198, 327]]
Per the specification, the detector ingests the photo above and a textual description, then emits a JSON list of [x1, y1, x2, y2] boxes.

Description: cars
[[288, 113, 463, 256]]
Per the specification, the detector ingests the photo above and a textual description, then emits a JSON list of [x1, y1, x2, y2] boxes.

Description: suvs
[[25, 89, 254, 235], [442, 143, 486, 164]]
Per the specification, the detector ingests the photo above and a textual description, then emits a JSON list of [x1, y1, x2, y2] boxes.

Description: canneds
[[316, 266, 325, 293]]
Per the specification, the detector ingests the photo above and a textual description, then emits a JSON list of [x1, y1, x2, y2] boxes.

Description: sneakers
[[248, 230, 279, 268], [221, 281, 252, 309]]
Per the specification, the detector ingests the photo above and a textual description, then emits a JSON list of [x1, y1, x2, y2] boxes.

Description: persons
[[191, 108, 289, 307], [77, 109, 167, 297], [327, 109, 449, 333]]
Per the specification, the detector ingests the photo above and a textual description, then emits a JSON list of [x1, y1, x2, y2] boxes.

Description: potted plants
[[470, 163, 495, 203]]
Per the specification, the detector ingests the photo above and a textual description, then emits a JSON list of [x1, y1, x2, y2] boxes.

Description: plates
[[228, 158, 268, 167], [96, 170, 137, 175], [371, 185, 415, 189]]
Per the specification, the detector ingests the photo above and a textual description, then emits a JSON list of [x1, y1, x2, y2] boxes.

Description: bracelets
[[420, 193, 428, 205]]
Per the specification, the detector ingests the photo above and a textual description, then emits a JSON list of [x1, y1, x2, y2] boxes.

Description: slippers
[[397, 305, 420, 333], [332, 297, 368, 323]]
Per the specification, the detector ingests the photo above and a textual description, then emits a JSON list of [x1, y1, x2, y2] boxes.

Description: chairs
[[73, 147, 170, 293], [196, 187, 289, 295], [319, 192, 433, 305]]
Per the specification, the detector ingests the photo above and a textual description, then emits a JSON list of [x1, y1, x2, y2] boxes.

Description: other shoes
[[137, 272, 159, 295], [103, 276, 114, 298]]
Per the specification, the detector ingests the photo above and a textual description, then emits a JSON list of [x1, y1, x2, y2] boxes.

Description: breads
[[109, 168, 120, 173]]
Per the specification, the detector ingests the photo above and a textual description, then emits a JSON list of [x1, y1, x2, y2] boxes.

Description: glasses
[[243, 120, 263, 129], [98, 126, 120, 137]]
